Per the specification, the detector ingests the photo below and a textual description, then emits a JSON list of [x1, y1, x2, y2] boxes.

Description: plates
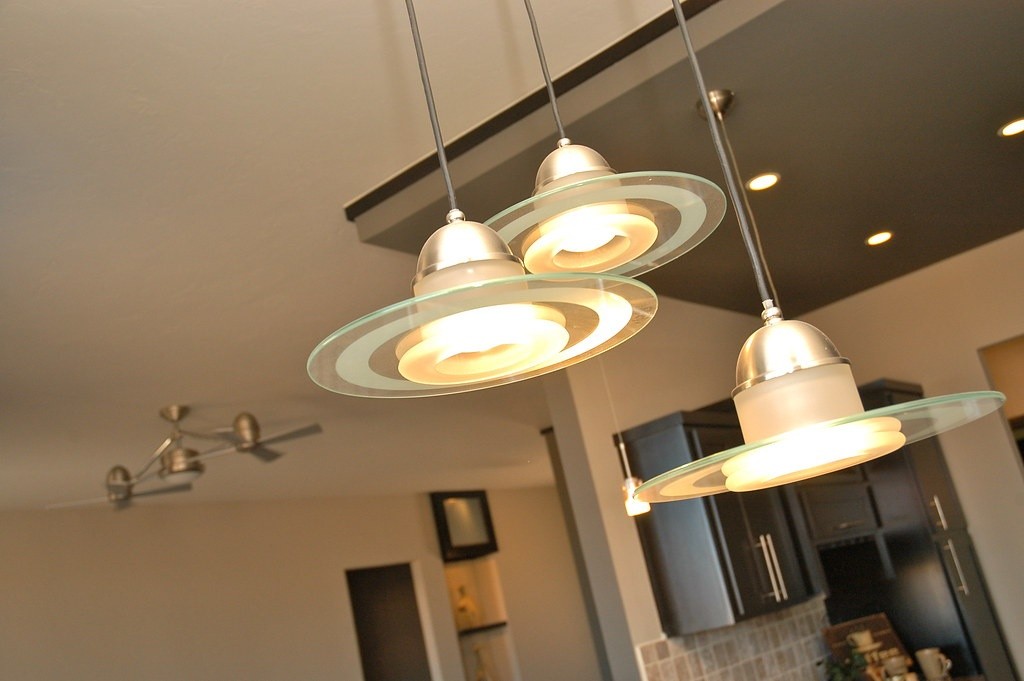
[[853, 641, 884, 654]]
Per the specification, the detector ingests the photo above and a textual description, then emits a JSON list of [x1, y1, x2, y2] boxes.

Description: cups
[[881, 656, 908, 681], [846, 629, 874, 647], [915, 647, 954, 681]]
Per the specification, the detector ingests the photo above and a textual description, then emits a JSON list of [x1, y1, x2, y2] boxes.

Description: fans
[[47, 402, 324, 513]]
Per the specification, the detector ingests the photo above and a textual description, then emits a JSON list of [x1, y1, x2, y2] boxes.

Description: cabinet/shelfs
[[818, 379, 1024, 681], [612, 409, 815, 639], [796, 452, 920, 549]]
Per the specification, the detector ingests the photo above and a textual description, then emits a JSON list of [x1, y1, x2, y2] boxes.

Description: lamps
[[597, 358, 651, 517], [305, 0, 658, 399], [631, 0, 1008, 503], [482, 0, 728, 292]]
